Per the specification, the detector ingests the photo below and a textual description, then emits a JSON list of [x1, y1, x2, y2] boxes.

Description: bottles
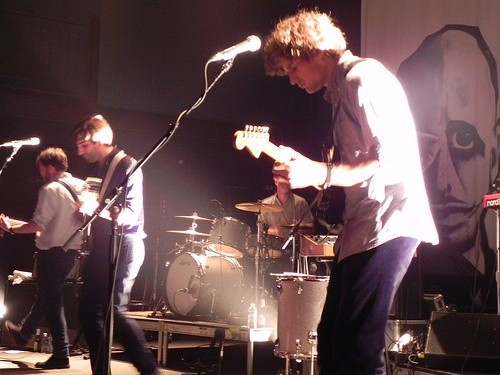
[[247, 303, 257, 329], [33, 328, 41, 352], [47, 337, 53, 352], [41, 333, 48, 353], [257, 299, 266, 328]]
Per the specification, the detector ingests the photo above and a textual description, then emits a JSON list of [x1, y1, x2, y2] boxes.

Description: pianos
[[484, 193, 500, 315]]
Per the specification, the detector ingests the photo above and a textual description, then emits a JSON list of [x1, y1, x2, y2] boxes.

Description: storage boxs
[[386, 319, 429, 351]]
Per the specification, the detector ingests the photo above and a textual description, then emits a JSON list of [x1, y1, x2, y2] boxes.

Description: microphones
[[209, 35, 261, 61], [179, 288, 196, 294], [3, 137, 40, 146]]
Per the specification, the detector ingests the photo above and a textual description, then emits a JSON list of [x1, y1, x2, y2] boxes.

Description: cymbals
[[234, 203, 283, 213], [281, 224, 315, 229], [165, 231, 209, 237], [175, 214, 213, 220]]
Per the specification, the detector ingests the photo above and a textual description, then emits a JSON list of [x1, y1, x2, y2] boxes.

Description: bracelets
[[319, 163, 332, 189]]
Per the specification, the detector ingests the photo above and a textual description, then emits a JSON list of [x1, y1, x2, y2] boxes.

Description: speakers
[[423, 310, 500, 371]]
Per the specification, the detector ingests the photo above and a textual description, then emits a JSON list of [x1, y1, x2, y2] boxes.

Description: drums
[[165, 252, 244, 317], [249, 234, 283, 258], [204, 217, 251, 259], [277, 272, 331, 359], [300, 234, 337, 257]]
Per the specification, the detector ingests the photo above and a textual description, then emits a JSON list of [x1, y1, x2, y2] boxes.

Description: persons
[[262, 5, 440, 375], [74, 113, 163, 375], [257, 184, 314, 249], [0, 147, 84, 370]]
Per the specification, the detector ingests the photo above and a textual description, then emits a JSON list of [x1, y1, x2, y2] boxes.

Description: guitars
[[234, 124, 344, 232], [0, 215, 28, 227]]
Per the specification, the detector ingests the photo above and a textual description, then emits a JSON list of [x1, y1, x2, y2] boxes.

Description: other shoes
[[5, 321, 27, 347], [141, 368, 162, 375], [35, 354, 68, 369]]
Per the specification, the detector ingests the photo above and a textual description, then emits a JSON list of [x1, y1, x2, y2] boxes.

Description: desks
[[4, 278, 86, 356]]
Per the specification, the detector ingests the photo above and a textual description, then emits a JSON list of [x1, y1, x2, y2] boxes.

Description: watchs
[[10, 227, 15, 235]]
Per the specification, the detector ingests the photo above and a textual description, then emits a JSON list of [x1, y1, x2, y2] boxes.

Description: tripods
[[226, 206, 278, 319]]
